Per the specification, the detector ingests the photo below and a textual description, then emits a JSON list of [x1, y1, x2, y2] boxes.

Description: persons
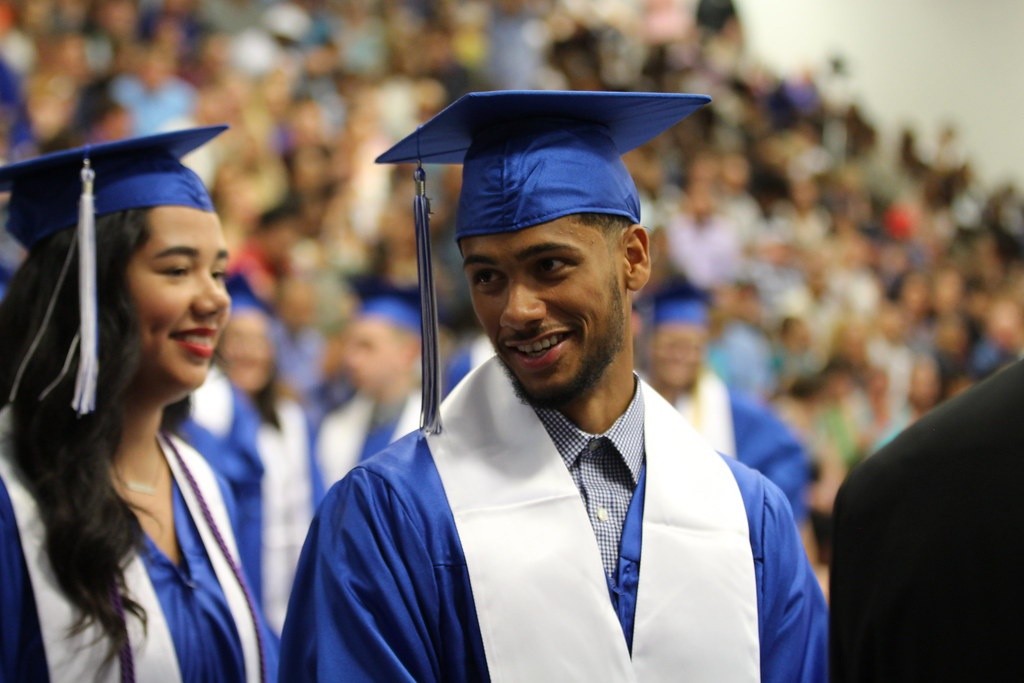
[[308, 270, 432, 502], [0, 0, 1024, 683], [0, 124, 281, 682], [171, 361, 263, 614], [831, 357, 1023, 683], [635, 271, 804, 527], [281, 93, 830, 682]]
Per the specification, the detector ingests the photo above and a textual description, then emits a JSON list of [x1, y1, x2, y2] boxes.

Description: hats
[[342, 272, 448, 338], [223, 272, 273, 316], [374, 90, 713, 438], [649, 272, 711, 329], [0, 122, 231, 417]]
